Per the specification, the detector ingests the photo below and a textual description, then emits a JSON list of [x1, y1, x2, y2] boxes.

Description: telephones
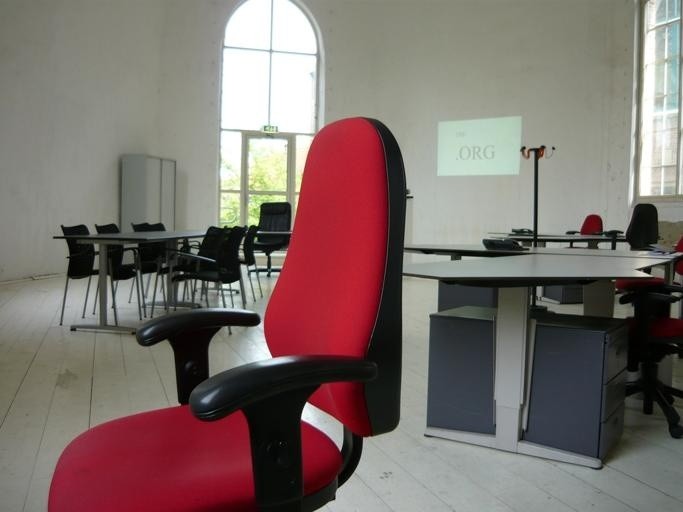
[[483, 238, 520, 251], [512, 228, 532, 234]]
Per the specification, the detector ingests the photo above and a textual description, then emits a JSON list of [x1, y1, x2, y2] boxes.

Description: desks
[[52, 229, 293, 335], [394, 232, 683, 470]]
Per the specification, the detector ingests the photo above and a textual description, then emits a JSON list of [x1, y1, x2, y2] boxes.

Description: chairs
[[59, 202, 265, 327], [565, 203, 683, 251], [248, 201, 292, 277], [46, 116, 408, 510]]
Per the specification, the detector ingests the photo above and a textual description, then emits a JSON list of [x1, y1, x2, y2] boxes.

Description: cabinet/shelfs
[[119, 154, 176, 234]]
